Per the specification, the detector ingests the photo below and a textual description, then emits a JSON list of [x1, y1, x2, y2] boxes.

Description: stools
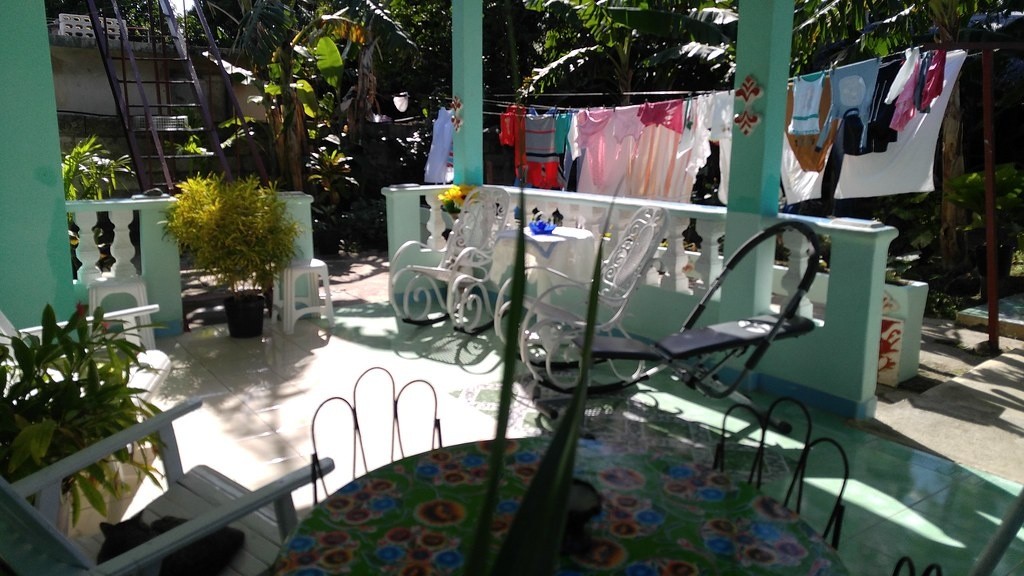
[[271, 257, 336, 335]]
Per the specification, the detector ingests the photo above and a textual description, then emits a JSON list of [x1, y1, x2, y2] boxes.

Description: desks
[[272, 437, 848, 575], [496, 226, 595, 341]]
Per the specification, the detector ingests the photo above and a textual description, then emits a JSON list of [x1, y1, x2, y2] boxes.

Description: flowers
[[438, 185, 479, 214]]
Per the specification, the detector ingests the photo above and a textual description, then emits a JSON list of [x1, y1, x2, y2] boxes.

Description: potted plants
[[159, 171, 306, 338]]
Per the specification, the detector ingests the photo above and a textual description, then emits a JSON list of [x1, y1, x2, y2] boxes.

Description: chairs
[[495, 205, 670, 389], [388, 186, 510, 336], [1, 398, 336, 575]]
